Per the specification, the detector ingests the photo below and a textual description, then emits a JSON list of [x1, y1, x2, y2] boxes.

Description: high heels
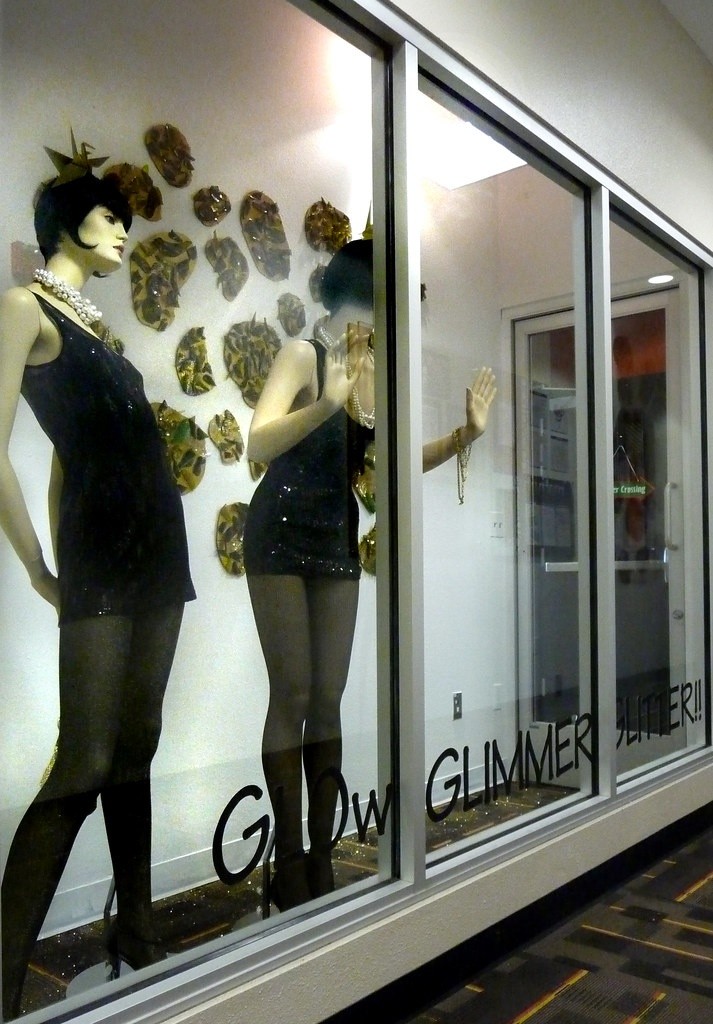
[[269, 847, 314, 913], [105, 919, 183, 981], [303, 847, 337, 898]]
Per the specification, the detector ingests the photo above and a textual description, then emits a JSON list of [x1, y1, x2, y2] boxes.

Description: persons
[[0, 172, 191, 1024], [244, 240, 497, 914]]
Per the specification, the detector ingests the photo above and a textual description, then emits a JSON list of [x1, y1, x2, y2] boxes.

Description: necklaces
[[33, 268, 102, 324], [318, 325, 375, 429]]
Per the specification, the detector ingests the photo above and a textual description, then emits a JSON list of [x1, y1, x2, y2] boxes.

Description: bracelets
[[452, 426, 472, 505]]
